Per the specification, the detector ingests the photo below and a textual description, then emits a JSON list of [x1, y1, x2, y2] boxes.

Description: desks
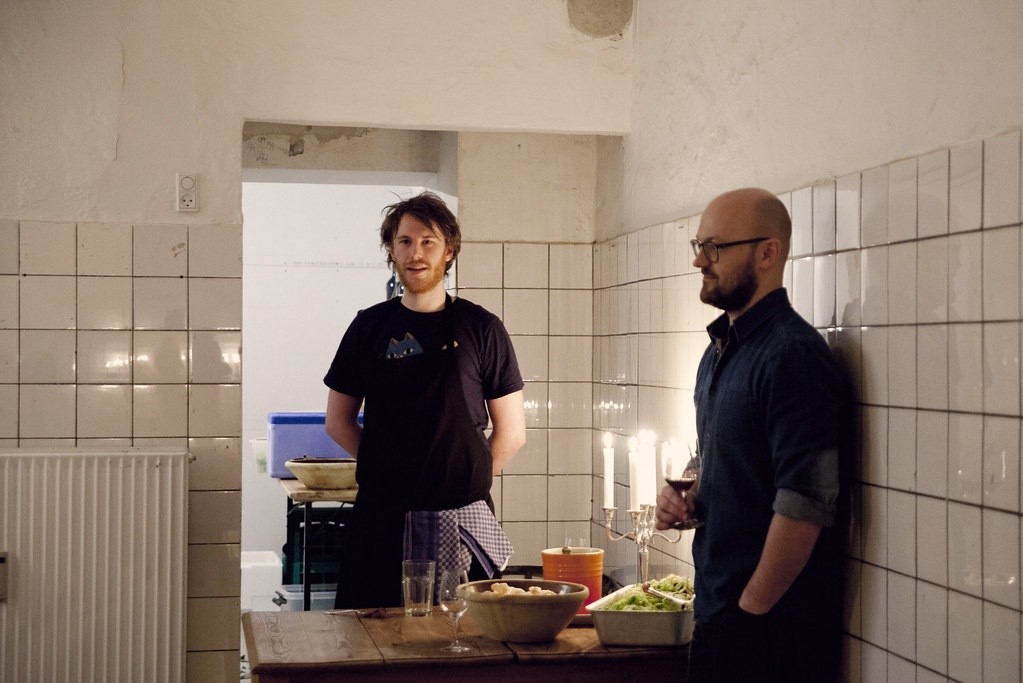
[[242, 604, 691, 683], [280, 478, 358, 610]]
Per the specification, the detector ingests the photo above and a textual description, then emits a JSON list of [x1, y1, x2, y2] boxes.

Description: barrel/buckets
[[541, 547, 604, 614]]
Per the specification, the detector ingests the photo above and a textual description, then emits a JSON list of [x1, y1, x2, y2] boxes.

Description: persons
[[319, 189, 528, 613], [650, 186, 864, 683]]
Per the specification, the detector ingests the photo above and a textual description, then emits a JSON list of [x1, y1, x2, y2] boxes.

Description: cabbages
[[602, 573, 696, 611]]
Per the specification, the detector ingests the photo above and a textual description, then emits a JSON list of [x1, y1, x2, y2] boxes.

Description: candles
[[626, 429, 657, 511], [602, 431, 615, 509]]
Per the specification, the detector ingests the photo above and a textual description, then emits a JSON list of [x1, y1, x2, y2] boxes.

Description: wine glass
[[439, 569, 472, 652], [661, 441, 700, 531]]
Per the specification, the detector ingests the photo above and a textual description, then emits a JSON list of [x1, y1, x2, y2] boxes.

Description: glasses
[[690, 236, 769, 262]]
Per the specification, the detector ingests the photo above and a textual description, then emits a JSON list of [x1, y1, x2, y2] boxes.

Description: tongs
[[642, 583, 697, 612]]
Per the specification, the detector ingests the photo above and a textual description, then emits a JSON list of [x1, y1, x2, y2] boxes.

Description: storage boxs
[[266, 413, 364, 480]]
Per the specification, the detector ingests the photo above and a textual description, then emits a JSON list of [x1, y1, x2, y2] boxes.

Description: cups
[[401, 559, 438, 617]]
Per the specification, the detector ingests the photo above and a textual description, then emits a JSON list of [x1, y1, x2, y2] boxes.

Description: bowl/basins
[[284, 457, 358, 489], [455, 579, 590, 642]]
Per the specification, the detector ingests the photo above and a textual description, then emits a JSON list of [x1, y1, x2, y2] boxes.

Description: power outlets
[[175, 172, 199, 212]]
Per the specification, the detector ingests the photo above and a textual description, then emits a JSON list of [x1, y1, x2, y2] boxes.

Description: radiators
[[0, 447, 198, 682]]
